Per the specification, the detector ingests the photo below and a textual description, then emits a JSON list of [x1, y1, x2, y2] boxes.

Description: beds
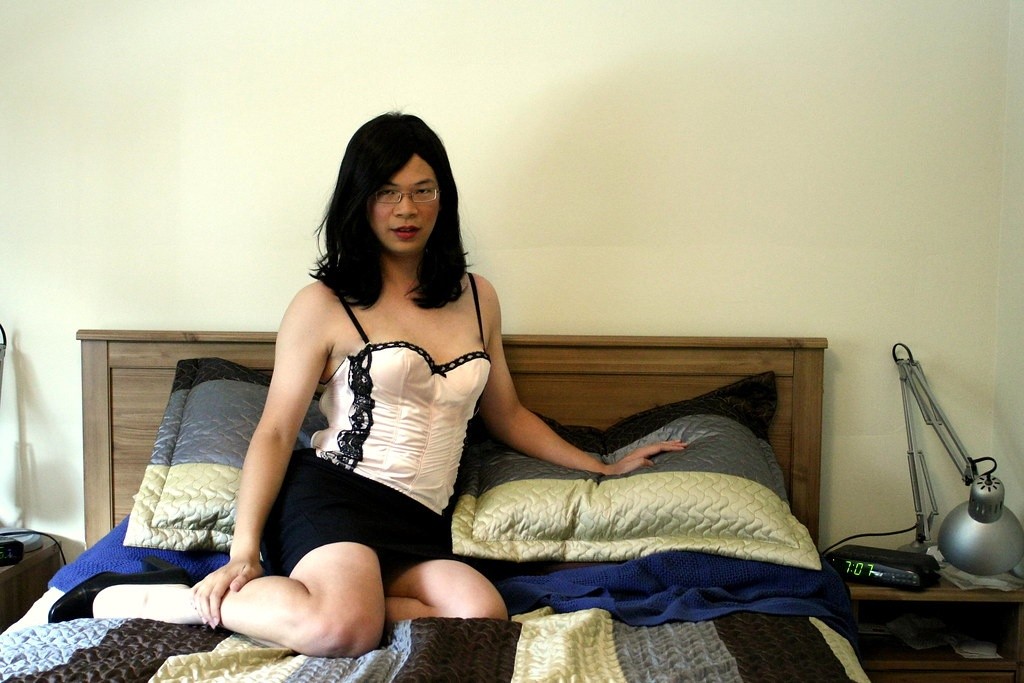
[[0, 330, 874, 683]]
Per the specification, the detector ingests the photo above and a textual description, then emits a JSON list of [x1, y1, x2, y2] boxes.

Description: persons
[[47, 112, 689, 658]]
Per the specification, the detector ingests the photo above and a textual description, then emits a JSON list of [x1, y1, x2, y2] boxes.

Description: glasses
[[371, 187, 443, 204]]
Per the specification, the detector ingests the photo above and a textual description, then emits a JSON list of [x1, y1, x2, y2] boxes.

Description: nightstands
[[0, 539, 63, 636], [848, 567, 1024, 682]]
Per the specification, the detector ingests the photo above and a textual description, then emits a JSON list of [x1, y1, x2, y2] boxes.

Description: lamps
[[890, 341, 1024, 576]]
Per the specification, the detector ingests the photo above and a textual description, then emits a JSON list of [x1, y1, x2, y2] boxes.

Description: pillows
[[452, 369, 821, 571], [122, 358, 330, 556]]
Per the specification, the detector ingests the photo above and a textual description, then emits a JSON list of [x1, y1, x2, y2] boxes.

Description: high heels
[[48, 554, 193, 624]]
